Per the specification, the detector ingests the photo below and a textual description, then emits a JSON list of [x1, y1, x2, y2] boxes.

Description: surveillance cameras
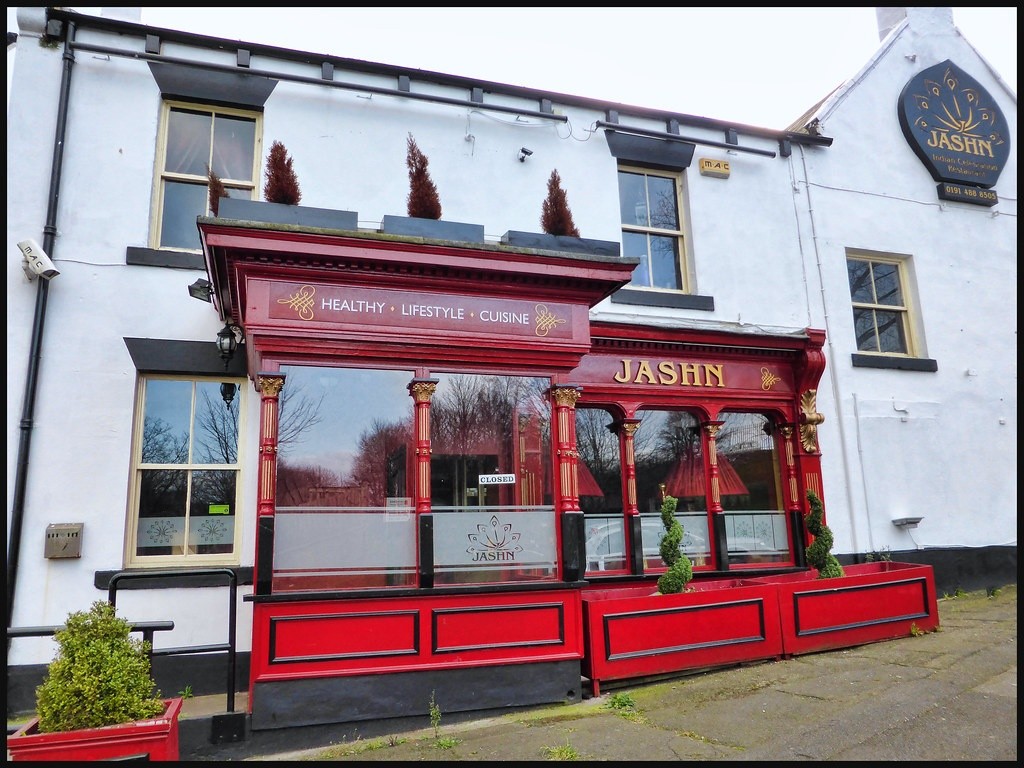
[[17, 239, 61, 281], [521, 148, 533, 155]]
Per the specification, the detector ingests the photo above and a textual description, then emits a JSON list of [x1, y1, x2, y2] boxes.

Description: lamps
[[216, 320, 244, 369], [187, 278, 216, 303], [220, 382, 236, 409], [892, 517, 924, 529]]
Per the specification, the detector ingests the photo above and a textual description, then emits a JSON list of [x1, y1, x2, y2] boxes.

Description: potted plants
[[742, 487, 939, 660], [582, 495, 782, 698], [8, 600, 184, 761]]
